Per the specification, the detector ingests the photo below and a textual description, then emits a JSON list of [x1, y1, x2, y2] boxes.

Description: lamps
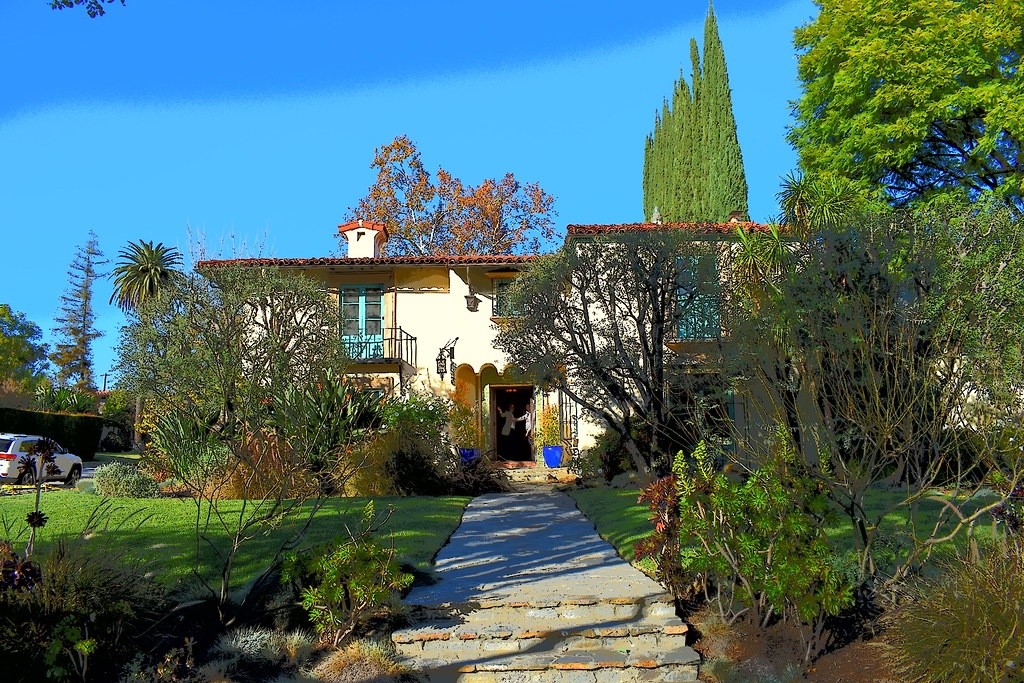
[[464, 286, 482, 312], [432, 336, 459, 381]]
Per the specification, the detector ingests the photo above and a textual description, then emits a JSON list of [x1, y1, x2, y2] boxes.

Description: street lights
[[100, 373, 110, 391]]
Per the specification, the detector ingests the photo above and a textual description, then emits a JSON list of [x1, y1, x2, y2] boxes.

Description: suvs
[[0, 432, 83, 485]]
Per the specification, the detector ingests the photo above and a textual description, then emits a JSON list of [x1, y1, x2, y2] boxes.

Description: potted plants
[[536, 402, 565, 468], [450, 402, 481, 464]]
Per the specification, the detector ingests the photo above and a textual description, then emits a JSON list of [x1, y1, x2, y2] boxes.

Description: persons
[[511, 403, 531, 446], [497, 404, 516, 460]]
